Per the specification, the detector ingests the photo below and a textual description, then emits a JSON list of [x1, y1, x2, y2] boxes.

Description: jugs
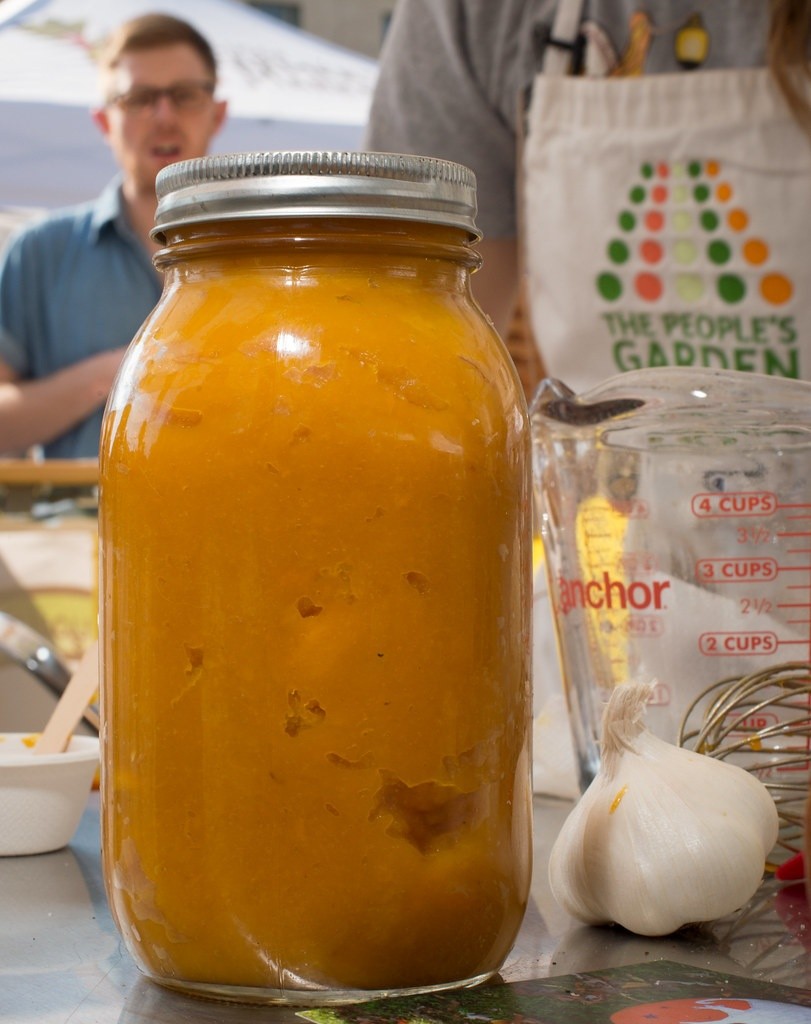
[[528, 366, 811, 886]]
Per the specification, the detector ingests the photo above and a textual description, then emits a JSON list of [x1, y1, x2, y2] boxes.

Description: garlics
[[547, 683, 778, 936]]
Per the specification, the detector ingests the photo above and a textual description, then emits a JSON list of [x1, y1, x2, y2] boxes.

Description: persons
[[1, 12, 227, 512], [367, 0, 811, 865]]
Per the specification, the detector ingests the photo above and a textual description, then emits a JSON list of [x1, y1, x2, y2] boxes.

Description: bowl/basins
[[0, 732, 100, 857]]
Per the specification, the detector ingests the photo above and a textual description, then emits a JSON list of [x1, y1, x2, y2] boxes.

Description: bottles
[[96, 151, 535, 1008]]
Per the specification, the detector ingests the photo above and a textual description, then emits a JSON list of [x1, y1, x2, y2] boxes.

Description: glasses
[[110, 78, 211, 113]]
